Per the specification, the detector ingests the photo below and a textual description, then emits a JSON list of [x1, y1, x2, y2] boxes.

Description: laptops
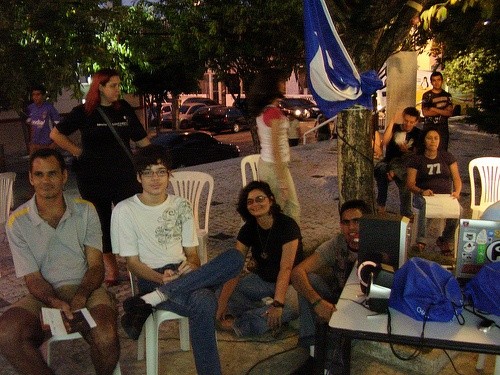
[[454, 219, 500, 285]]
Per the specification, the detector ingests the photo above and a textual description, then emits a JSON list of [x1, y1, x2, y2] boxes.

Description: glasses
[[137, 169, 168, 177], [247, 194, 270, 205]]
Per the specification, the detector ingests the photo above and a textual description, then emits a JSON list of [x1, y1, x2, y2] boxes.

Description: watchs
[[273, 300, 284, 309]]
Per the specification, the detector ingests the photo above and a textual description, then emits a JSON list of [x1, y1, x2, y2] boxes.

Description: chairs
[[39, 333, 122, 375], [0, 171, 17, 226], [468, 157, 500, 220], [169, 171, 215, 266], [240, 154, 261, 225], [130, 270, 219, 375], [410, 191, 458, 259]]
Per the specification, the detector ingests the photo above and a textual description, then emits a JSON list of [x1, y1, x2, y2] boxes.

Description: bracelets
[[312, 298, 322, 306]]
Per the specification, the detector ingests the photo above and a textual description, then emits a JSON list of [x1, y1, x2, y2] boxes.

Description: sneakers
[[120, 294, 157, 340]]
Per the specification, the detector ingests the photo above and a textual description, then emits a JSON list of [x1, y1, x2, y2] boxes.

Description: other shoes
[[216, 317, 236, 331], [436, 236, 453, 253], [407, 212, 415, 223], [378, 205, 386, 215], [291, 353, 325, 375], [411, 242, 425, 252], [271, 321, 289, 339]]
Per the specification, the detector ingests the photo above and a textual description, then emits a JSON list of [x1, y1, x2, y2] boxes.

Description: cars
[[145, 96, 255, 133], [134, 130, 241, 171], [279, 95, 321, 121]]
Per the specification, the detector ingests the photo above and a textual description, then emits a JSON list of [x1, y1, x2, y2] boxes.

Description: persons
[[115, 145, 245, 375], [374, 107, 422, 221], [249, 69, 301, 226], [290, 199, 372, 375], [25, 87, 59, 156], [422, 72, 454, 150], [0, 147, 121, 375], [49, 68, 152, 287], [220, 181, 305, 337], [407, 128, 462, 254], [314, 114, 337, 142]]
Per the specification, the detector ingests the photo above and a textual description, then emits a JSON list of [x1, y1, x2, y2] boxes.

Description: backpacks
[[385, 256, 464, 360], [461, 262, 500, 329]]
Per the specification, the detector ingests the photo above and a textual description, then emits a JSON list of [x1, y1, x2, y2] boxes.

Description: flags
[[303, 0, 383, 119]]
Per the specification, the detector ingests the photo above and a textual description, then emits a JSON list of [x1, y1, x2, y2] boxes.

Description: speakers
[[359, 215, 410, 300]]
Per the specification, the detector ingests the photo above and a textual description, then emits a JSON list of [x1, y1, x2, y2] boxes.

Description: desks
[[328, 259, 500, 375]]
